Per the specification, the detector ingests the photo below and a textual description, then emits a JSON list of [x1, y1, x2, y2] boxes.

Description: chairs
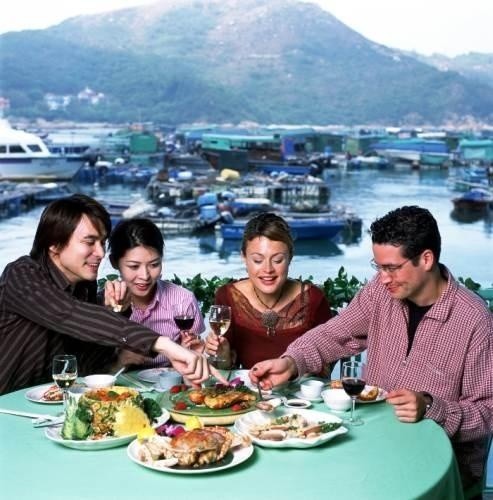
[[471, 288, 492, 491]]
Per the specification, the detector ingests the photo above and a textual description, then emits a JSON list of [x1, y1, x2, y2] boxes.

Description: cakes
[[170, 426, 233, 468]]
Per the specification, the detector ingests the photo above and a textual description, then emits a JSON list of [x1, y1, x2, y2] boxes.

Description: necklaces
[[254, 287, 283, 337]]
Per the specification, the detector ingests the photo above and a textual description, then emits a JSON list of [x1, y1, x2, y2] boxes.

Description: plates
[[46, 407, 174, 451], [126, 426, 255, 476], [357, 386, 388, 403], [295, 390, 324, 402], [24, 384, 65, 403], [137, 367, 175, 382], [157, 384, 265, 426], [232, 406, 352, 450], [151, 382, 168, 392]]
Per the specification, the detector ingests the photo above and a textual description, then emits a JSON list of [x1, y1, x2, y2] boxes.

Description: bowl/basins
[[285, 397, 312, 409], [321, 388, 354, 411]]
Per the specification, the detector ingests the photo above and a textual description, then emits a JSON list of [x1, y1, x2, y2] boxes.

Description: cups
[[301, 378, 323, 398], [158, 372, 182, 389], [83, 373, 116, 386]]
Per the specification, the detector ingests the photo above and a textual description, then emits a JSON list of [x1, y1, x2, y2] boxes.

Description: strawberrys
[[180, 384, 188, 392], [232, 404, 240, 412], [174, 402, 186, 411], [171, 385, 179, 393]]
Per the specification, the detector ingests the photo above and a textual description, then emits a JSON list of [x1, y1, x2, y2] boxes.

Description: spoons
[[251, 368, 274, 413]]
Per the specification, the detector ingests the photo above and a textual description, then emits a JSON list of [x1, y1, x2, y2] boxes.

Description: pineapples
[[81, 402, 143, 435]]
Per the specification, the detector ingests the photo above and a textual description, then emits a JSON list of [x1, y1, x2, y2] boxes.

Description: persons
[[96, 218, 205, 371], [249, 206, 493, 491], [0, 193, 231, 396], [205, 212, 332, 379]]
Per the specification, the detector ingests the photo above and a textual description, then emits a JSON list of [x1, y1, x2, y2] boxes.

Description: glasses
[[368, 253, 418, 274]]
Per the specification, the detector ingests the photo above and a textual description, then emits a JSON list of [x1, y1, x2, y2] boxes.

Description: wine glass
[[172, 300, 230, 366], [340, 360, 369, 427], [49, 353, 78, 416]]
